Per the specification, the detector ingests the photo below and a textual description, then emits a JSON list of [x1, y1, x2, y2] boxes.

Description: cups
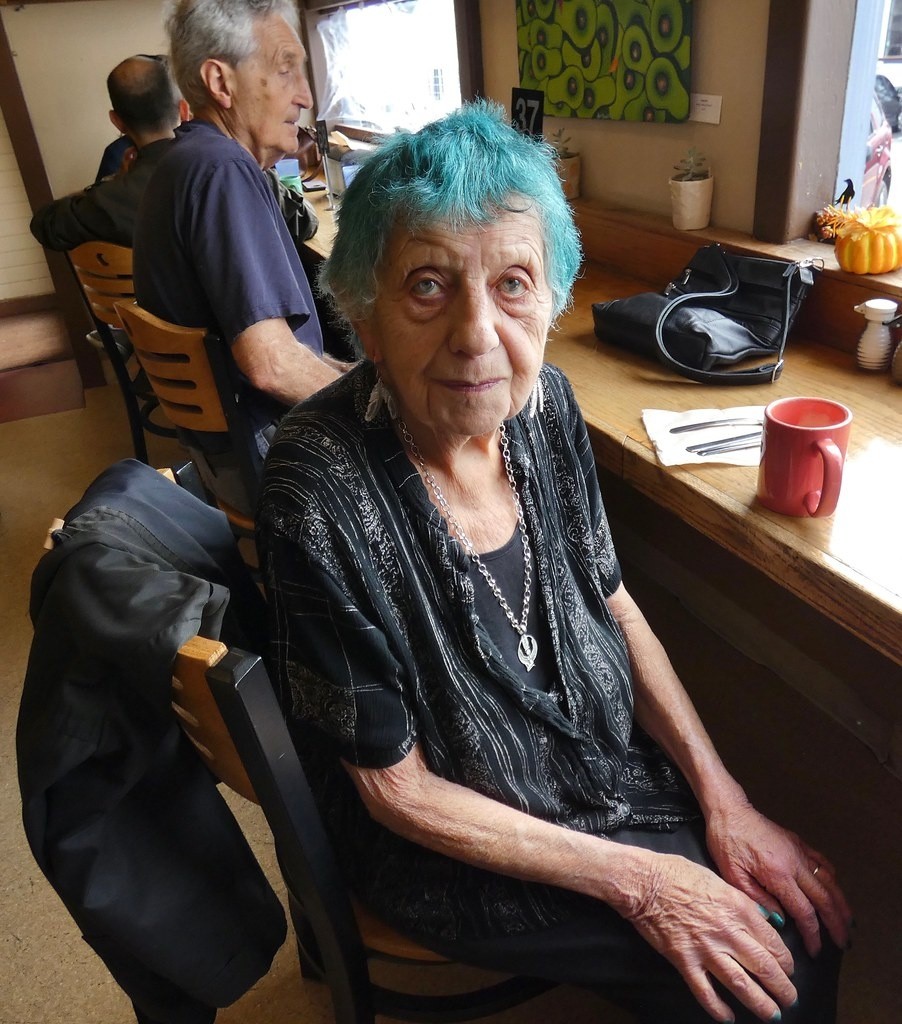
[[342, 165, 364, 188], [756, 397, 854, 517], [275, 159, 300, 176], [279, 174, 303, 196]]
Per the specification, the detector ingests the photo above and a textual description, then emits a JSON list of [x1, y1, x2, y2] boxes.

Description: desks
[[298, 166, 901, 663]]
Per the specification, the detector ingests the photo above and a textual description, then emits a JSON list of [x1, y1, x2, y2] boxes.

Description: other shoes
[[86, 323, 133, 360]]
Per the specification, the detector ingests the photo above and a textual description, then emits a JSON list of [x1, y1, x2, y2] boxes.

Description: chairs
[[77, 456, 564, 1024], [62, 239, 180, 467], [112, 299, 272, 607]]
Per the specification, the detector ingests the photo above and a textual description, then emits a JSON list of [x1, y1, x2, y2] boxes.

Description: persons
[[28, 1, 365, 514], [249, 98, 854, 1024]]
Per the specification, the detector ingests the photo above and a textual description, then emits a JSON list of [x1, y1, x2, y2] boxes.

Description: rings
[[812, 863, 821, 875]]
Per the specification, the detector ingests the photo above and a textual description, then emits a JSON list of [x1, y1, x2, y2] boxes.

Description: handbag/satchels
[[591, 241, 825, 385], [281, 124, 323, 182]]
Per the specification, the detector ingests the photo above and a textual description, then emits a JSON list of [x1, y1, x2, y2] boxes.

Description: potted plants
[[550, 128, 582, 201], [670, 147, 715, 231]]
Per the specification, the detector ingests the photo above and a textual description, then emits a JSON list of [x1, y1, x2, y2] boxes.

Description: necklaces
[[375, 370, 546, 673]]
